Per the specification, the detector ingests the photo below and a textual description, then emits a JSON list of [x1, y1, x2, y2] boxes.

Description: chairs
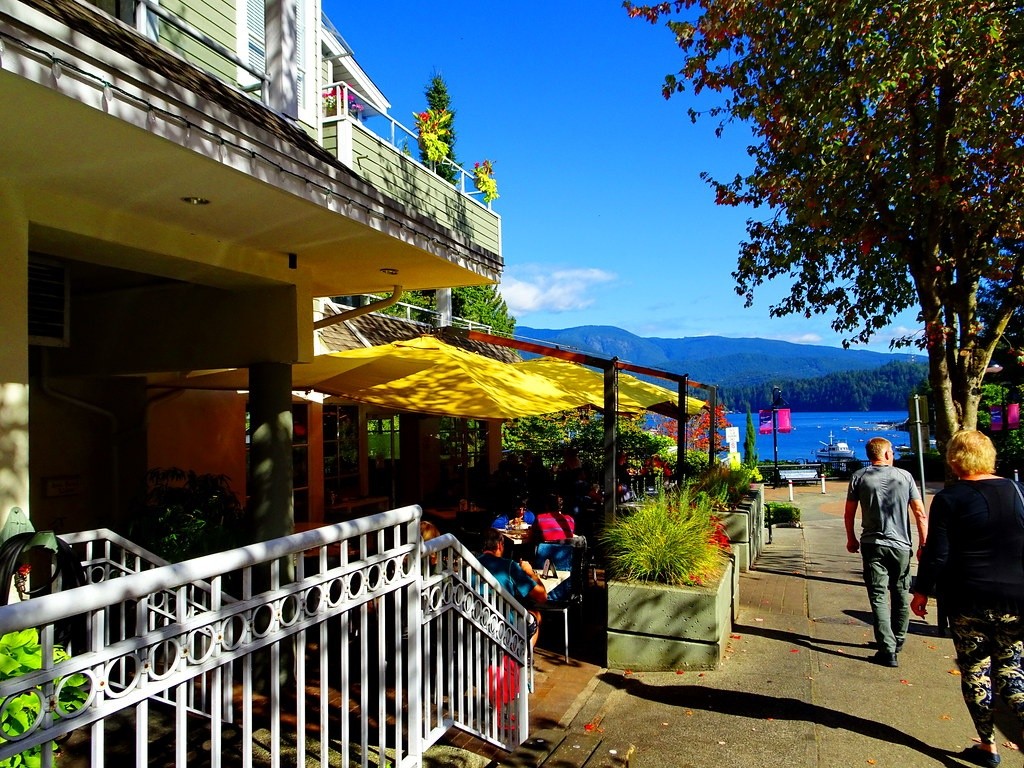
[[456, 511, 495, 555], [531, 542, 574, 663]]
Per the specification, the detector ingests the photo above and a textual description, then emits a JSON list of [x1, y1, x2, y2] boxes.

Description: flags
[[777, 408, 792, 433], [990, 405, 1003, 431], [1006, 403, 1020, 430], [759, 409, 773, 434]]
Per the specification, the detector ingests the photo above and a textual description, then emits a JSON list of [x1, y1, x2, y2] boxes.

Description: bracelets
[[918, 546, 925, 550]]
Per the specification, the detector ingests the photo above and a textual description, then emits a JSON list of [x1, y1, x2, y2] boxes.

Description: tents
[[146, 334, 706, 425]]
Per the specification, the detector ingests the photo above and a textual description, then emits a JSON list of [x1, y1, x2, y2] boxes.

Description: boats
[[815, 430, 855, 458]]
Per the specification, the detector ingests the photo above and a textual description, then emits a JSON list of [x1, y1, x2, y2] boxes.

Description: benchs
[[774, 467, 821, 488]]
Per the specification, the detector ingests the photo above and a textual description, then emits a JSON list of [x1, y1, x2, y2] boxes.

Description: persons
[[843, 437, 929, 667], [385, 453, 670, 666], [911, 427, 1024, 768]]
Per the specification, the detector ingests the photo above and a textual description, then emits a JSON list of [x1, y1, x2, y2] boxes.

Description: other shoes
[[874, 651, 898, 668], [963, 744, 1002, 768], [895, 638, 906, 652]]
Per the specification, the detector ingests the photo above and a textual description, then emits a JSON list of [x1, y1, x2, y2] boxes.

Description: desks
[[533, 569, 571, 592]]
